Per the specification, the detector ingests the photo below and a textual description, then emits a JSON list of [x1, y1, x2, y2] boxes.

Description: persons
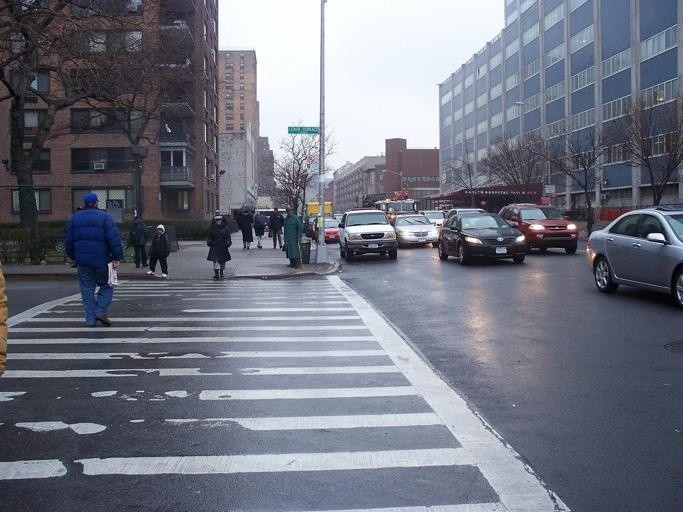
[[127, 216, 148, 268], [268, 207, 285, 249], [207, 216, 232, 279], [254, 212, 270, 248], [67, 204, 85, 268], [146, 224, 171, 278], [239, 209, 254, 249], [212, 210, 226, 224], [283, 207, 304, 268], [64, 193, 121, 327]]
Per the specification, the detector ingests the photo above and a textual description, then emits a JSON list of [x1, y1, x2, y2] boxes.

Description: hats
[[84, 193, 99, 204], [156, 225, 165, 234]]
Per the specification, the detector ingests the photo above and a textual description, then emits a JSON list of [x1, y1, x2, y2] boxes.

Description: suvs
[[337, 210, 398, 260], [498, 202, 579, 255]]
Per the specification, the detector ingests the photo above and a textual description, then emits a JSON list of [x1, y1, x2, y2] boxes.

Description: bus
[[254, 207, 302, 224]]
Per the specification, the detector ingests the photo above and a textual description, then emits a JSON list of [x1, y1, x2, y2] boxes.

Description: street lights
[[515, 99, 545, 152], [382, 168, 403, 215], [130, 143, 149, 219]]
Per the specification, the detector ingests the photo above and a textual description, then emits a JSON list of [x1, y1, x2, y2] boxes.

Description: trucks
[[302, 200, 334, 216]]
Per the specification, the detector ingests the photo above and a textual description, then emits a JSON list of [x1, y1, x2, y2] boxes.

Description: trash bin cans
[[299, 239, 311, 264]]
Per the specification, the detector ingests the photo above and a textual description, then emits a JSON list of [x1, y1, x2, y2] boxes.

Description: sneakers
[[146, 271, 154, 274], [97, 315, 112, 326], [129, 230, 140, 243], [161, 273, 168, 278]]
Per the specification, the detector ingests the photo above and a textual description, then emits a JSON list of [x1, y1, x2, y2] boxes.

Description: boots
[[214, 269, 224, 278]]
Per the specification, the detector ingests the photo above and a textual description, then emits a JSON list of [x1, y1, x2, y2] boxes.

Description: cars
[[442, 208, 487, 228], [438, 212, 527, 266], [296, 211, 343, 244], [420, 210, 444, 229], [391, 214, 440, 247], [588, 203, 683, 308]]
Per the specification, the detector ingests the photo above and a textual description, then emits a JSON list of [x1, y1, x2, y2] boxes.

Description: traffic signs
[[288, 127, 322, 135]]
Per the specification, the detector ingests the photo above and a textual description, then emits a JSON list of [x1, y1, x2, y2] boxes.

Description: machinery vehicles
[[362, 188, 418, 217]]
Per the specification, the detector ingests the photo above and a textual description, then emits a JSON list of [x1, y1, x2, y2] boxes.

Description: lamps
[[219, 170, 225, 177], [2, 158, 9, 171]]
